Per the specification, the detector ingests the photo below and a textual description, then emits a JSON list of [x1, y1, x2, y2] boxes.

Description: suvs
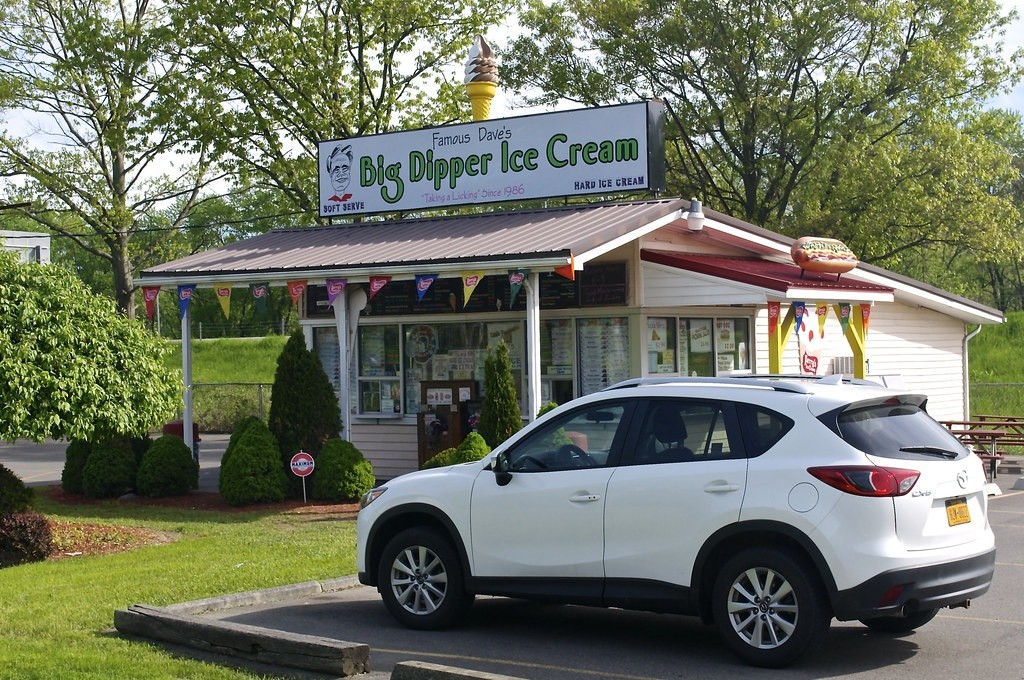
[[353, 371, 998, 667]]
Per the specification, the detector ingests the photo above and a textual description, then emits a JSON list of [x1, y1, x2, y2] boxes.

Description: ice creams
[[464, 33, 499, 121]]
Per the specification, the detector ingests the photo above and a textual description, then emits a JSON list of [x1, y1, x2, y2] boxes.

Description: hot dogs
[[791, 236, 857, 273]]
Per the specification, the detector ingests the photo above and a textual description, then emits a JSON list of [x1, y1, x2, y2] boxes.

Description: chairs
[[645, 408, 695, 462]]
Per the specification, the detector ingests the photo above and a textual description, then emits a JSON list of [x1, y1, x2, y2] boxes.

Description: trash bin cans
[[162, 420, 201, 461], [416, 379, 479, 469]]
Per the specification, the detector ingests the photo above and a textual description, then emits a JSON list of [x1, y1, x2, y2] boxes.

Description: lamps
[[678, 201, 704, 231]]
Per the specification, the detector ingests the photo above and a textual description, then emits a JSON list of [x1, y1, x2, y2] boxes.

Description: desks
[[936, 414, 1024, 456]]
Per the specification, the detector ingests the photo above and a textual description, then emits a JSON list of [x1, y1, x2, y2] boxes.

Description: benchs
[[953, 433, 1024, 482]]
